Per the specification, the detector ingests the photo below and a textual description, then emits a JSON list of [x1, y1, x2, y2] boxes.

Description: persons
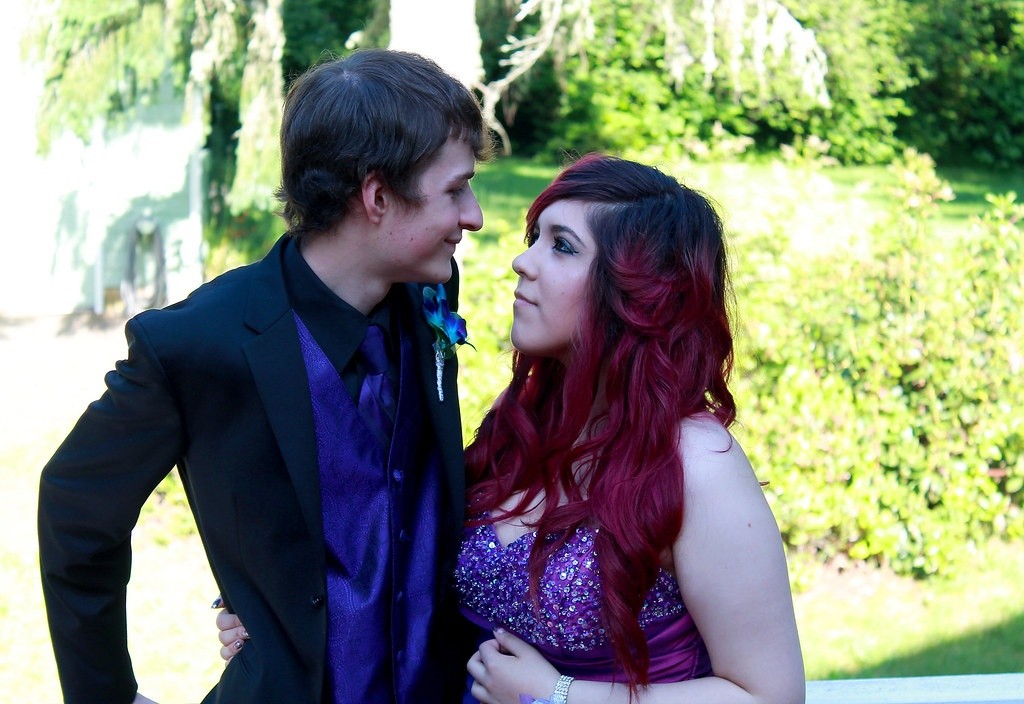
[[211, 150, 807, 704], [37, 47, 502, 704]]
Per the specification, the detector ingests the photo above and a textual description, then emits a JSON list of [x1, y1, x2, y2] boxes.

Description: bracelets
[[550, 674, 574, 704]]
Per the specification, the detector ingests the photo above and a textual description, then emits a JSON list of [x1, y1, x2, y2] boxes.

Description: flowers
[[420, 284, 476, 362]]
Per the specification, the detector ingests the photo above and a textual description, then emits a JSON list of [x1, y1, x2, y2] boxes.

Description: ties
[[358, 326, 401, 464]]
